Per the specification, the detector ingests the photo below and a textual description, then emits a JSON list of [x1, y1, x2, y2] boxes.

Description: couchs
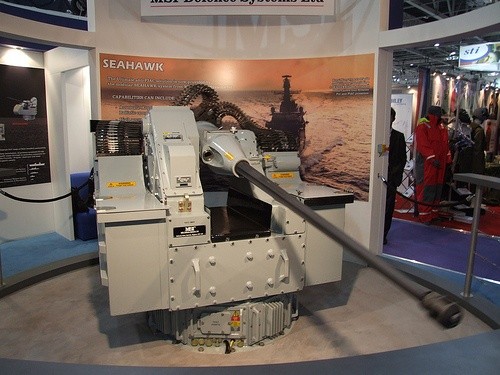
[[70, 171, 98, 242]]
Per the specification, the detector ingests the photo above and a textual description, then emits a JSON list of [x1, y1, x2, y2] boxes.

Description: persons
[[412, 103, 450, 226], [381, 106, 408, 245], [471, 107, 491, 215], [448, 107, 473, 215]]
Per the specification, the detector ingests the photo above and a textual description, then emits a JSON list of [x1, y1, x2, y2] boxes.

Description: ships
[[264, 74, 310, 155]]
